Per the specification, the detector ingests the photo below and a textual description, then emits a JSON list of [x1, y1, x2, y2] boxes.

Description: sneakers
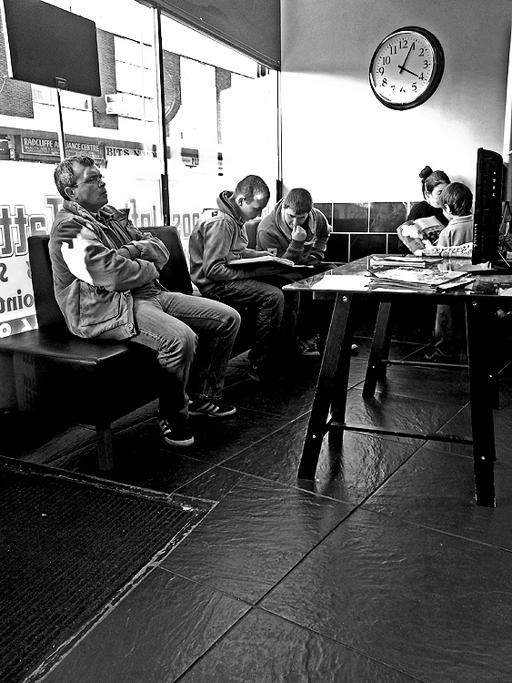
[[188, 398, 237, 417], [423, 347, 458, 364], [298, 338, 321, 356], [156, 417, 195, 447]]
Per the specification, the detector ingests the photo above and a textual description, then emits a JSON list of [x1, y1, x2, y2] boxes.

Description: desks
[[282, 253, 512, 509]]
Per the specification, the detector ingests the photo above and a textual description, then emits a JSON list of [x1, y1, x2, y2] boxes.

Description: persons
[[405, 166, 453, 257], [49, 155, 241, 446], [188, 175, 300, 382], [257, 186, 360, 354], [436, 181, 474, 249]]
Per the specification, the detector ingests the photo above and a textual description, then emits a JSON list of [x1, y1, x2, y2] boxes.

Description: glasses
[[71, 176, 105, 188]]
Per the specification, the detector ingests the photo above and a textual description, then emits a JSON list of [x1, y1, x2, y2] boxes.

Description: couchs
[[0, 225, 194, 475]]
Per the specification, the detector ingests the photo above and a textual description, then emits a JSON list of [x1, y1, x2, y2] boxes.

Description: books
[[370, 255, 476, 294], [228, 255, 318, 273]]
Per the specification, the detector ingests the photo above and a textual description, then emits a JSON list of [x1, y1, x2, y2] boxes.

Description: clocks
[[368, 27, 444, 109]]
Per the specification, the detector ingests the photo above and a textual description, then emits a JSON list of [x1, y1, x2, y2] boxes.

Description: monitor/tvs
[[472, 147, 509, 267]]
